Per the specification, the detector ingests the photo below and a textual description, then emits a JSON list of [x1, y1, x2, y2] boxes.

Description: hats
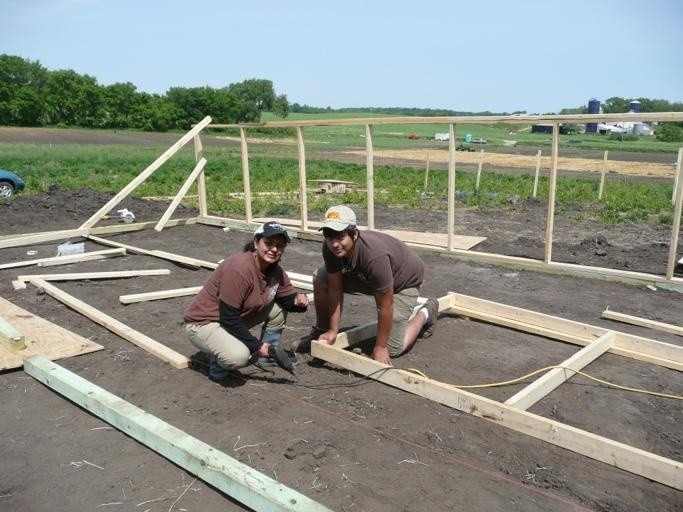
[[318, 206, 356, 231], [253, 222, 292, 243]]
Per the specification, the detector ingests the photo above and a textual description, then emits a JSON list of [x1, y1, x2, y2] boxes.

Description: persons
[[183, 221, 309, 381], [289, 206, 439, 369]]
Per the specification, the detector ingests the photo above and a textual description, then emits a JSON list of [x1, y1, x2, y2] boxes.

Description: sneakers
[[257, 356, 300, 367], [420, 298, 439, 338], [210, 356, 227, 381], [291, 326, 326, 351]]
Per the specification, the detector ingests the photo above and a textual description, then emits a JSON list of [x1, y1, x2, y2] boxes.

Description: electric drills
[[254, 347, 303, 384]]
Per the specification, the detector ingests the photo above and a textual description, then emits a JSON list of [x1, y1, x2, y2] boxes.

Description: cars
[[470, 139, 487, 144], [0, 169, 24, 198]]
